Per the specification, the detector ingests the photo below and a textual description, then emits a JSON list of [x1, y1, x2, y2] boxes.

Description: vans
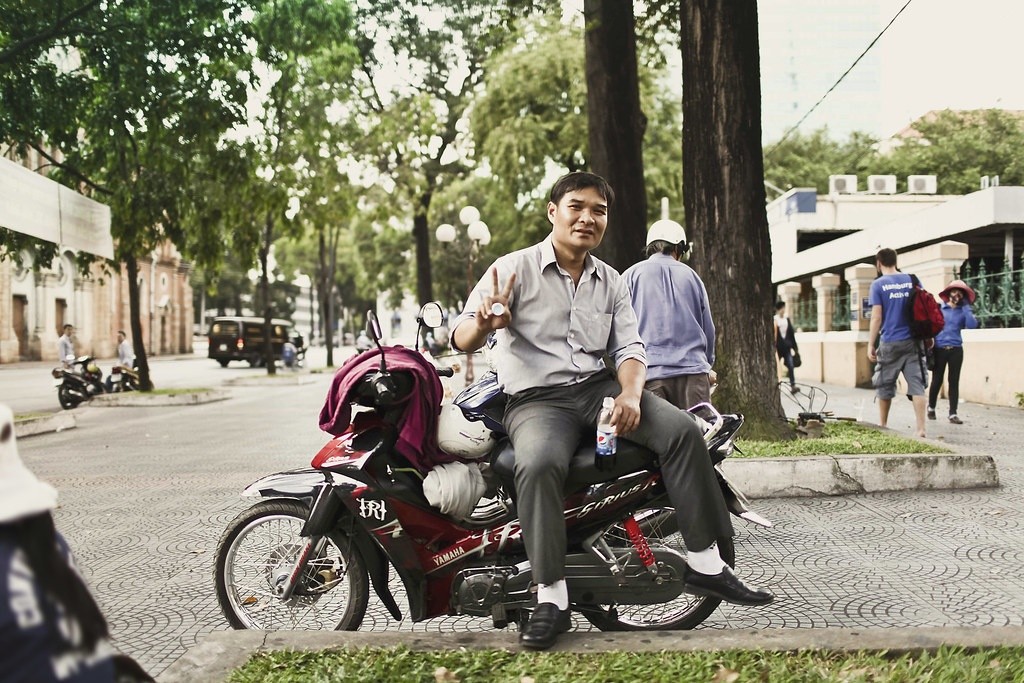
[[208, 317, 305, 368]]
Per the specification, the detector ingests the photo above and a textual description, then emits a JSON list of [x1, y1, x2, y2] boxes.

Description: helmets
[[438, 404, 499, 458], [646, 219, 688, 247]]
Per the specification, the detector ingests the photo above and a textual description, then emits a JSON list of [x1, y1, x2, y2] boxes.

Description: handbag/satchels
[[793, 353, 802, 367], [925, 348, 935, 371]]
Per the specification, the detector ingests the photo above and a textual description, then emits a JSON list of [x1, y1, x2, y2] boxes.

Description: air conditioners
[[829, 175, 857, 194], [866, 174, 897, 194], [906, 175, 938, 195]]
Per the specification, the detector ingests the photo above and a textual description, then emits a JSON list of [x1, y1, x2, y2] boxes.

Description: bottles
[[595, 397, 617, 471]]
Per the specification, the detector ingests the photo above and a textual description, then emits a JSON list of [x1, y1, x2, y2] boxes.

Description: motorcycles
[[52, 350, 108, 411], [213, 304, 772, 631], [108, 358, 155, 392]]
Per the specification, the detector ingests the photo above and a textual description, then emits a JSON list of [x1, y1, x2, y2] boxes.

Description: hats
[[939, 280, 976, 305]]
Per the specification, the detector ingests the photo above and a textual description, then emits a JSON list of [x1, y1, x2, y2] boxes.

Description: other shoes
[[790, 385, 800, 395], [926, 405, 937, 421], [949, 414, 963, 424]]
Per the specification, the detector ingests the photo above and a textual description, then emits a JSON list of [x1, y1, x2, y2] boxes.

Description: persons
[[773, 301, 800, 394], [867, 247, 936, 438], [927, 279, 978, 424], [57, 323, 76, 368], [355, 330, 374, 353], [617, 218, 718, 417], [283, 338, 298, 367], [105, 330, 134, 393], [449, 171, 776, 652]]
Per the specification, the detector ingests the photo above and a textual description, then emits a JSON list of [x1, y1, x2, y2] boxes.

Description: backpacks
[[905, 274, 945, 340]]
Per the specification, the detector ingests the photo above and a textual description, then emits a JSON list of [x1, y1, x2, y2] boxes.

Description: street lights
[[435, 207, 492, 386]]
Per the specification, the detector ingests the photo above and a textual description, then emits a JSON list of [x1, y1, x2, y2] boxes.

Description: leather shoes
[[519, 602, 572, 649], [683, 563, 773, 606]]
